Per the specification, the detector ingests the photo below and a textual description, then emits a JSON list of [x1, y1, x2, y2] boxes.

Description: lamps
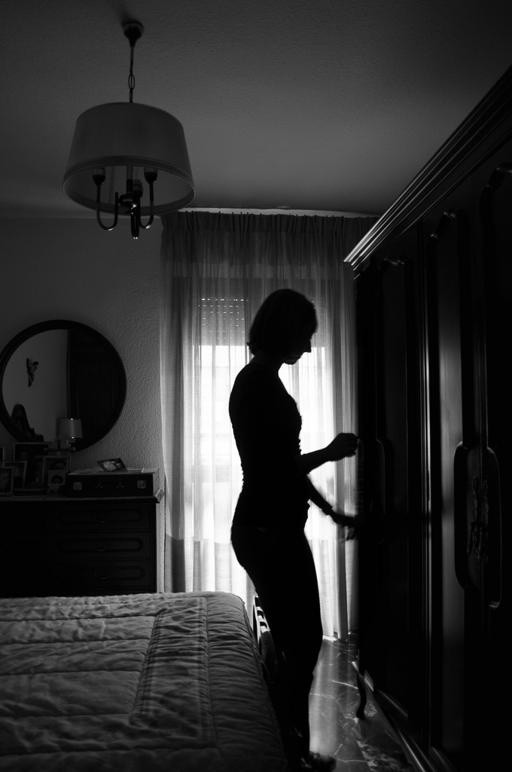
[[60, 15, 198, 241]]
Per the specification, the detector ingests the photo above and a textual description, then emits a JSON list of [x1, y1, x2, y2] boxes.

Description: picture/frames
[[0, 442, 72, 497]]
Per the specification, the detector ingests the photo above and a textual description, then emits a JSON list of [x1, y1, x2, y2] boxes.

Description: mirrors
[[0, 319, 127, 453]]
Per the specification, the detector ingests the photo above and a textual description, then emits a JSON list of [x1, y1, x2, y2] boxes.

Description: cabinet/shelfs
[[0, 488, 166, 597]]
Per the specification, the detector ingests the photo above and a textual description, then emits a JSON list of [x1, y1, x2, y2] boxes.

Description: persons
[[225, 288, 363, 771]]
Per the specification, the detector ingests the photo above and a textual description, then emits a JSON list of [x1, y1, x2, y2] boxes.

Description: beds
[[0, 591, 292, 770]]
